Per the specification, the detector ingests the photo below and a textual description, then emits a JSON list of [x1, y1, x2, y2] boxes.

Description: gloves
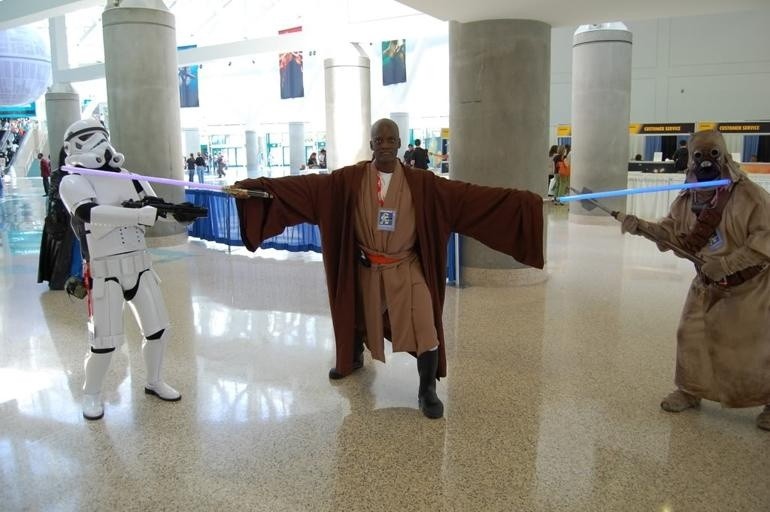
[[621, 214, 649, 235], [701, 255, 727, 283]]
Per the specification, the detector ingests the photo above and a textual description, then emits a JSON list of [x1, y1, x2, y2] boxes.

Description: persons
[[187, 152, 225, 183], [751, 155, 758, 161], [307, 150, 327, 170], [38, 152, 51, 196], [224, 119, 544, 419], [2, 116, 29, 167], [672, 140, 688, 170], [622, 130, 770, 432], [403, 138, 431, 169], [635, 154, 641, 160], [548, 145, 571, 205], [58, 119, 204, 421]]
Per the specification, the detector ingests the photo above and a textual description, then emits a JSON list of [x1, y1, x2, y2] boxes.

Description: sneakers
[[660, 389, 701, 413]]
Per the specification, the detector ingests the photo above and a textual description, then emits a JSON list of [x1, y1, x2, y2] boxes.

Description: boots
[[417, 348, 443, 418], [142, 338, 181, 401], [83, 351, 113, 420], [328, 331, 364, 379]]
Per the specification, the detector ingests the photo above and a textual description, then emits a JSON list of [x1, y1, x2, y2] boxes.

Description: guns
[[118, 196, 210, 224]]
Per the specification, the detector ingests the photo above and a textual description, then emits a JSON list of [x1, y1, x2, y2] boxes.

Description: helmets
[[63, 118, 125, 170]]
